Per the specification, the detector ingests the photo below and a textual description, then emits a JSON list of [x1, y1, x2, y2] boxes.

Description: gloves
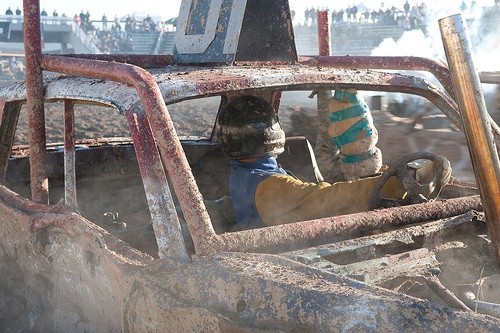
[[415, 154, 452, 186]]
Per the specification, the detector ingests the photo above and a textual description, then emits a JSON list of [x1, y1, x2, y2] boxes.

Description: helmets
[[216, 96, 285, 160]]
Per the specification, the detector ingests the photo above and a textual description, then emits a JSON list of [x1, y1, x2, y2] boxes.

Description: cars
[[1, 0, 500, 333]]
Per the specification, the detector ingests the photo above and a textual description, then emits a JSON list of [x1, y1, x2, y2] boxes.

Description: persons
[[1, 0, 500, 59], [193, 96, 453, 236]]
